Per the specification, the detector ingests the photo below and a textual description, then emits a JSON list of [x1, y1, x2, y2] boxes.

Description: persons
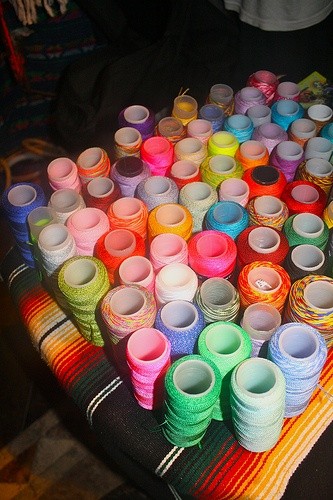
[[0, 0, 332, 155]]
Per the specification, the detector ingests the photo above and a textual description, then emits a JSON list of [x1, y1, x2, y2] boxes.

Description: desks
[[0, 225, 332, 500]]
